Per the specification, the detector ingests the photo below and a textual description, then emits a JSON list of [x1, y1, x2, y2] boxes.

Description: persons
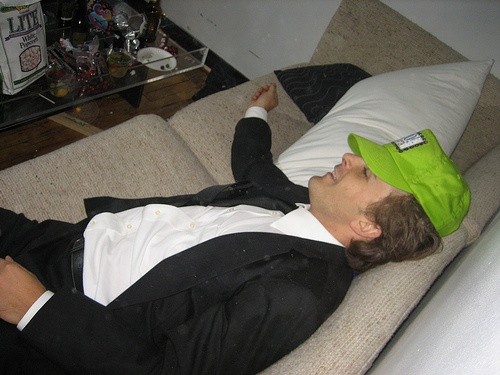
[[1, 83, 469, 375]]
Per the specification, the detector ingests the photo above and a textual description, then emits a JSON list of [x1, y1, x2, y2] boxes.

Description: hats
[[347, 128, 471, 238]]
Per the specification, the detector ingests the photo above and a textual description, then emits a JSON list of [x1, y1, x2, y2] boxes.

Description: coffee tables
[[0, 0, 209, 133]]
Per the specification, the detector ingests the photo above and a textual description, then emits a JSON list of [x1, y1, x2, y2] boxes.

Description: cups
[[107, 48, 130, 79], [45, 63, 74, 97]]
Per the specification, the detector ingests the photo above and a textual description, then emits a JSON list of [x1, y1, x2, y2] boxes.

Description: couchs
[[0, 0, 499, 375]]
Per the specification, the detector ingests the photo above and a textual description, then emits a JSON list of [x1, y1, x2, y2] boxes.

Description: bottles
[[56, 0, 75, 27], [142, 0, 162, 43], [71, 0, 88, 48]]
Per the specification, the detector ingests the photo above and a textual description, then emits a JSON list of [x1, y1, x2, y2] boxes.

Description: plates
[[136, 47, 177, 71]]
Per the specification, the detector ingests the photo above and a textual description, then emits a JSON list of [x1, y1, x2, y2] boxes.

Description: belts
[[70, 238, 85, 298]]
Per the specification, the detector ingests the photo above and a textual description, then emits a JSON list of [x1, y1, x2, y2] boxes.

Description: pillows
[[272, 62, 372, 126], [273, 60, 494, 188]]
